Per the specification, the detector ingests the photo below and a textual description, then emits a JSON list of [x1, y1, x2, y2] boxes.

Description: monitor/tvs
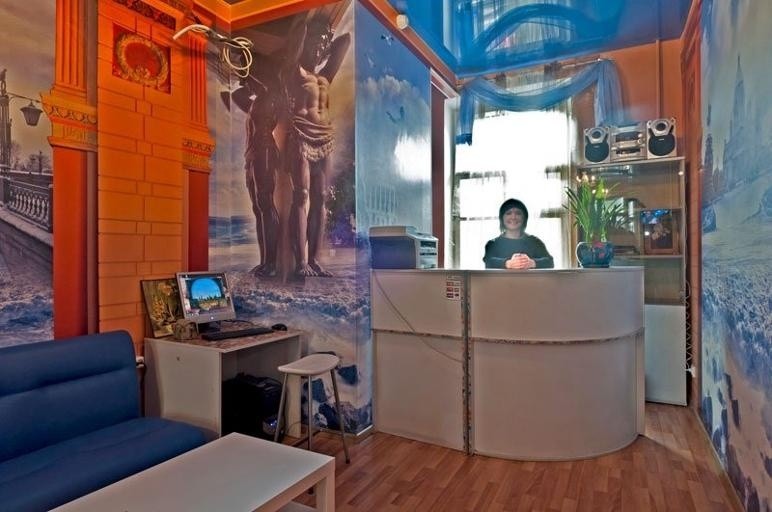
[[175, 270, 237, 333]]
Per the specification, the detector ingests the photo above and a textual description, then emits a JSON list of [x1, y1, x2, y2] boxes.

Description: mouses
[[271, 323, 288, 331]]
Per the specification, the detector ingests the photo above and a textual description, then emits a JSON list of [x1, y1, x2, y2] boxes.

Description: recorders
[[582, 116, 678, 165]]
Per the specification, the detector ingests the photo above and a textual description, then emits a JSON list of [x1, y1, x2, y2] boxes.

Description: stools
[[273, 353, 351, 495]]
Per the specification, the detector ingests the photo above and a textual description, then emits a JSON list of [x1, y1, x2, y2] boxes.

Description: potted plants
[[560, 175, 642, 268]]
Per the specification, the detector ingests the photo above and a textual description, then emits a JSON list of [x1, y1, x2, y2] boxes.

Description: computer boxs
[[222, 370, 287, 443]]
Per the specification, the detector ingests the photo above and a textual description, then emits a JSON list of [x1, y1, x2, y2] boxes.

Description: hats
[[499, 199, 527, 219]]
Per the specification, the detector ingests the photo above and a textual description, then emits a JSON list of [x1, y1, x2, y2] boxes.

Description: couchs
[[0, 329, 210, 512]]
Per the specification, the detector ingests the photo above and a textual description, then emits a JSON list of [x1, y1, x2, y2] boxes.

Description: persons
[[483, 199, 554, 268], [232, 52, 282, 278], [278, 15, 350, 276]]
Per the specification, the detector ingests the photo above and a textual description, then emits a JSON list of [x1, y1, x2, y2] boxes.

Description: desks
[[143, 317, 304, 439]]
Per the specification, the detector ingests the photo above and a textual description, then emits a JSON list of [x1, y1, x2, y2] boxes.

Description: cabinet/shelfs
[[567, 151, 690, 408]]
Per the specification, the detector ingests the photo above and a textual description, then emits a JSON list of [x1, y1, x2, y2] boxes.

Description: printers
[[369, 225, 439, 269]]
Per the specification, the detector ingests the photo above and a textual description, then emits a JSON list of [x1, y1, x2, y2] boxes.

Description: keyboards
[[201, 327, 273, 342]]
